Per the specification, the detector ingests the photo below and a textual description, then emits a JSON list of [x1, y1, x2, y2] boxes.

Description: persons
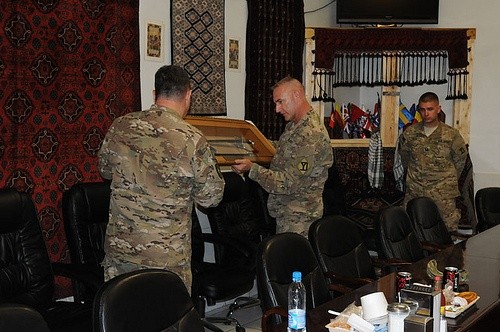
[[98, 65, 225, 298], [233, 76, 333, 241], [399, 91, 467, 243]]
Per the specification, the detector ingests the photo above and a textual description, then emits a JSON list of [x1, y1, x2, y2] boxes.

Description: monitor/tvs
[[335, 0, 440, 25]]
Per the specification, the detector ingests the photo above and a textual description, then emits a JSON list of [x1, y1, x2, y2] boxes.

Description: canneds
[[442, 267, 459, 293], [396, 272, 412, 299]]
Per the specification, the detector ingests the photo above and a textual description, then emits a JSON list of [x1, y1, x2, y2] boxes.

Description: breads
[[455, 291, 478, 302]]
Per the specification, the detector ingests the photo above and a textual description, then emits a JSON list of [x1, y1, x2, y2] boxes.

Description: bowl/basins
[[360, 292, 388, 322]]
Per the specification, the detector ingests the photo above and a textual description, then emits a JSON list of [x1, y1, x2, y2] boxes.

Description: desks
[[265, 224, 500, 332]]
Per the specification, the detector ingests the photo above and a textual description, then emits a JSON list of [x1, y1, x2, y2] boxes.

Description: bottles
[[387, 297, 419, 332], [433, 276, 446, 319], [287, 272, 306, 332]]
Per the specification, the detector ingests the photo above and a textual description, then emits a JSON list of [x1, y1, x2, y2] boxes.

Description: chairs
[[308, 215, 375, 298], [257, 232, 354, 332], [92, 269, 205, 332], [61, 182, 111, 306], [406, 197, 454, 259], [0, 189, 103, 332], [191, 204, 253, 332], [475, 187, 500, 234], [375, 207, 424, 273], [207, 171, 262, 325]]
